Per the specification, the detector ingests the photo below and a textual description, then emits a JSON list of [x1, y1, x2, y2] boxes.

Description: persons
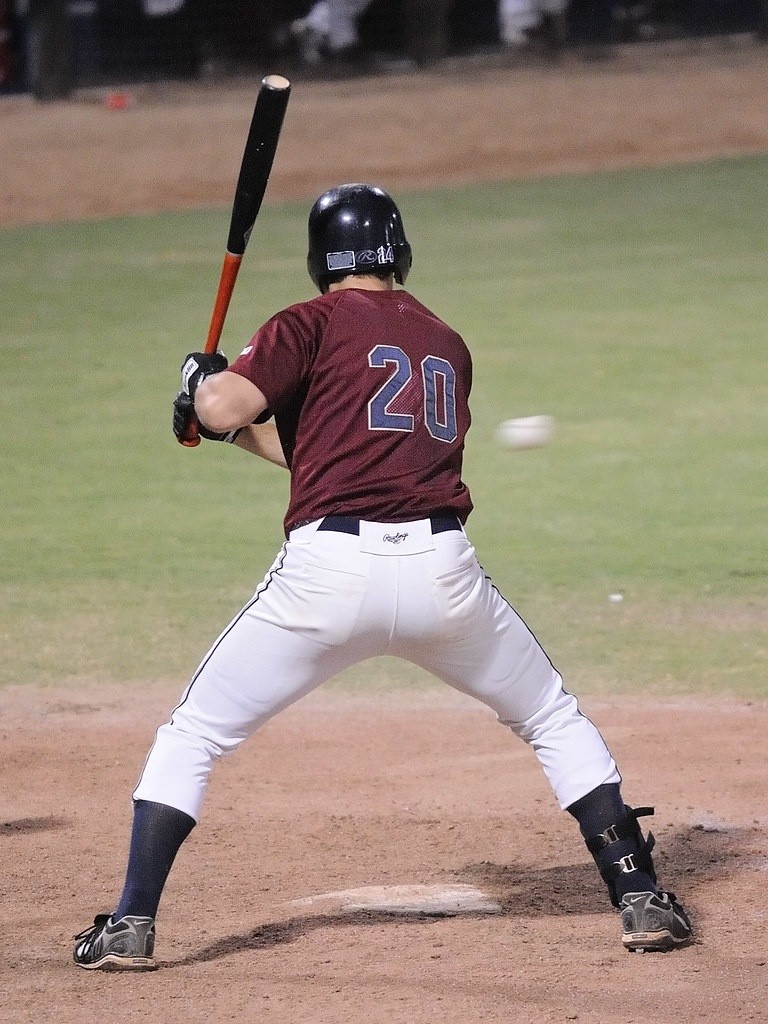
[[72, 182, 692, 973], [19, 0, 566, 102]]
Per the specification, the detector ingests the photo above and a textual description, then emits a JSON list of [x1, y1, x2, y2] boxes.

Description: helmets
[[307, 183, 412, 294]]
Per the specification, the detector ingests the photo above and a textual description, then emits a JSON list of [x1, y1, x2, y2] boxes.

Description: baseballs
[[500, 414, 546, 451]]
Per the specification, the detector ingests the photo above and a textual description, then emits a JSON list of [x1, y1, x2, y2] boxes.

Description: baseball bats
[[179, 76, 295, 451]]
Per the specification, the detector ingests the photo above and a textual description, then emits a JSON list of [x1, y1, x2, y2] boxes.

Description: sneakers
[[71, 911, 156, 971], [621, 888, 692, 954]]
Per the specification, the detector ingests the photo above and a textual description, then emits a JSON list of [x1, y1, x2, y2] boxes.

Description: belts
[[316, 515, 462, 536]]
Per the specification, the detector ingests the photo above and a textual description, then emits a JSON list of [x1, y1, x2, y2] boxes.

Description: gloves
[[172, 391, 243, 447], [180, 349, 228, 403]]
